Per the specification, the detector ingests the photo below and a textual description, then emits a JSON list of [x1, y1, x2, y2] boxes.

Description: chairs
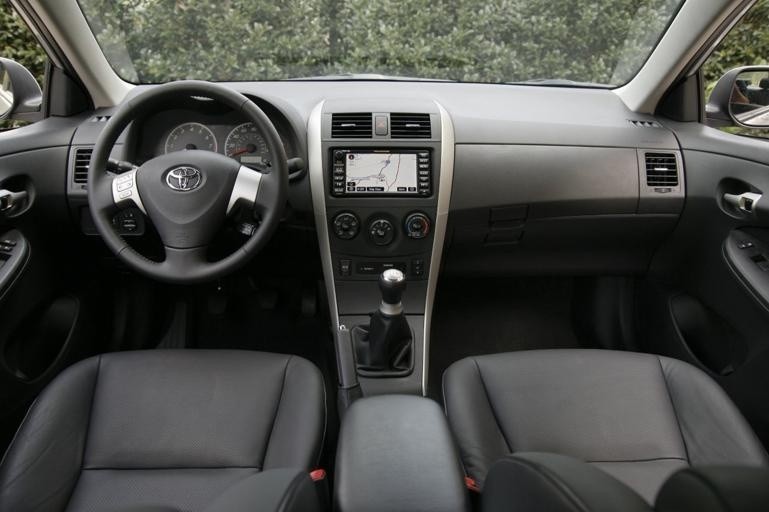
[[441, 347, 769, 512], [750, 78, 769, 104], [0, 347, 330, 512], [735, 79, 751, 102]]
